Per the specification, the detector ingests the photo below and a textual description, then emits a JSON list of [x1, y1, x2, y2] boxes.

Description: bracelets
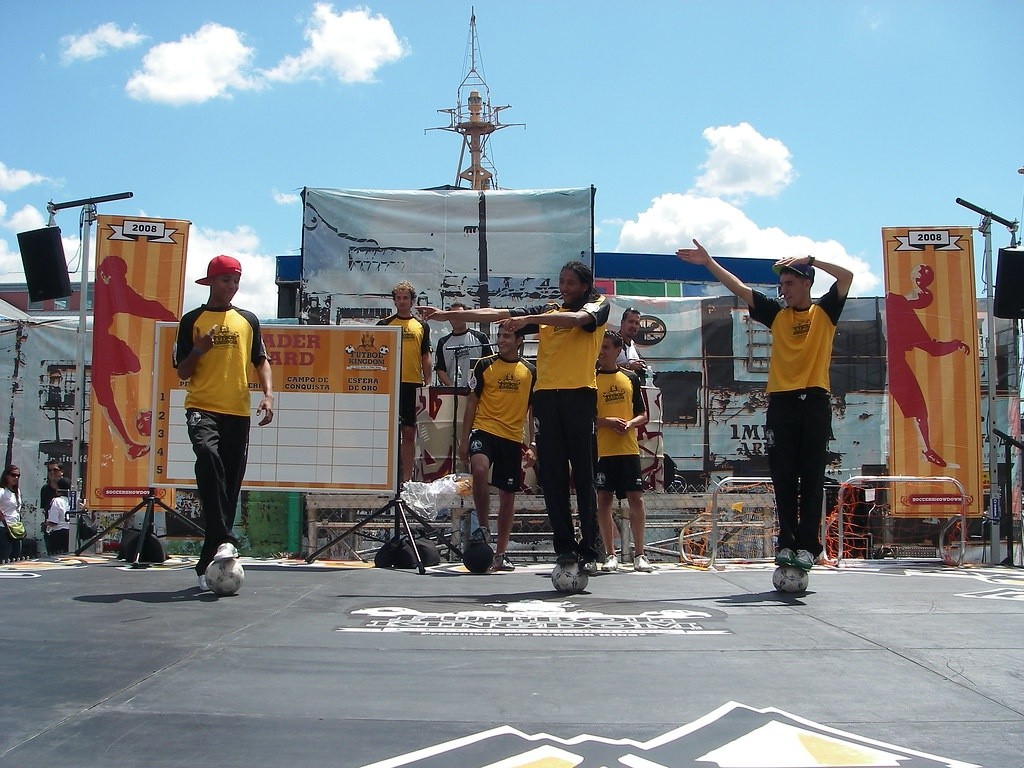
[[807, 254, 815, 265], [529, 440, 537, 448], [191, 346, 204, 356]]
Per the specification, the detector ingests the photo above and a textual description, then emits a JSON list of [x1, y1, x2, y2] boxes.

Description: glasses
[[623, 306, 640, 317], [48, 468, 61, 472], [9, 473, 21, 479]]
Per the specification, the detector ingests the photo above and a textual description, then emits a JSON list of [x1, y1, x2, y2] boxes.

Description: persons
[[171, 253, 275, 591], [43, 477, 72, 555], [675, 238, 854, 570], [375, 280, 434, 483], [434, 300, 495, 389], [0, 464, 24, 563], [415, 259, 610, 574], [615, 307, 648, 387], [458, 320, 538, 574], [40, 456, 68, 555], [593, 329, 656, 573]]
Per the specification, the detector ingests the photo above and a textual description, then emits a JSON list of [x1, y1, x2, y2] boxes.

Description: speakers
[[993, 246, 1024, 319], [17, 227, 73, 302]]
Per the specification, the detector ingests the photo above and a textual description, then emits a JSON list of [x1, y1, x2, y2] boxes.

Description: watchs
[[625, 362, 631, 369]]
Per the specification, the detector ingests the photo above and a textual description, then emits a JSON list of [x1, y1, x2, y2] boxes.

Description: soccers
[[773, 564, 809, 593], [204, 557, 244, 595], [550, 562, 588, 594]]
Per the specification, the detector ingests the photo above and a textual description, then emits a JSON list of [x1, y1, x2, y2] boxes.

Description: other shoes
[[556, 550, 578, 561], [125, 443, 151, 461], [583, 559, 598, 572], [601, 554, 619, 571], [634, 554, 653, 572]]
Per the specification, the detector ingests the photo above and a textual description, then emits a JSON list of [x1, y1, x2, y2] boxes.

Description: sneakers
[[466, 527, 493, 542], [793, 549, 815, 572], [774, 548, 797, 567], [198, 574, 211, 591], [494, 553, 515, 571], [212, 542, 239, 562]]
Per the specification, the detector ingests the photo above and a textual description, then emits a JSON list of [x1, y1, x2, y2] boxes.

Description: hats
[[194, 255, 242, 285], [57, 477, 71, 492], [772, 260, 816, 284]]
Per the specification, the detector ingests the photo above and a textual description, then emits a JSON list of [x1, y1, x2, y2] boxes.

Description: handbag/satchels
[[77, 513, 98, 542], [6, 522, 28, 540], [117, 526, 166, 563]]
